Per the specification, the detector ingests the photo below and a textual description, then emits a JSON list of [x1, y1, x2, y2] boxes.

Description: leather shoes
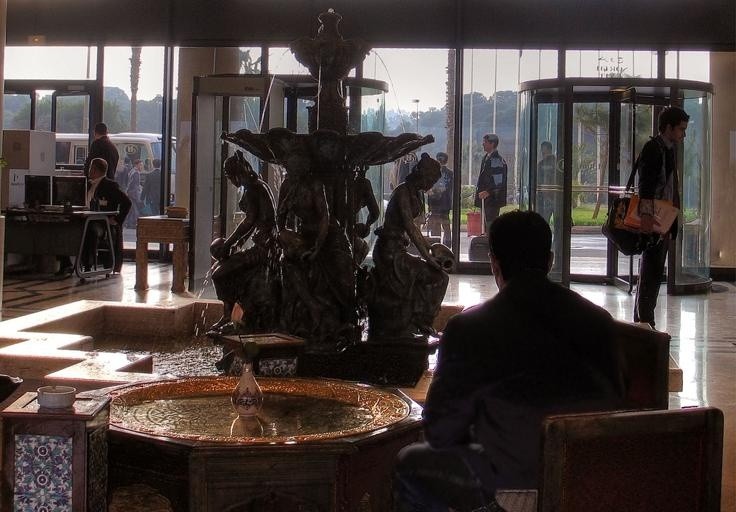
[[82, 265, 91, 278], [55, 266, 74, 275]]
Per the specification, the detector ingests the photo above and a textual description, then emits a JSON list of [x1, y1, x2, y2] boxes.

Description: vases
[[231, 363, 265, 415]]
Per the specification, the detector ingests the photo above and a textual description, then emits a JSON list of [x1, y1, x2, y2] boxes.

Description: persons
[[473, 134, 505, 231], [83, 123, 119, 180], [391, 209, 627, 512], [633, 107, 690, 331], [39, 157, 132, 275], [126, 159, 143, 225], [428, 152, 454, 248], [140, 159, 161, 215], [116, 158, 131, 191], [206, 149, 441, 337], [390, 149, 417, 191], [537, 141, 557, 228]]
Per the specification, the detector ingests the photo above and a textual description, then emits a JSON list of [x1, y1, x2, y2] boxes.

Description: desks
[[134, 215, 222, 296], [0, 391, 112, 511], [7, 207, 120, 283]]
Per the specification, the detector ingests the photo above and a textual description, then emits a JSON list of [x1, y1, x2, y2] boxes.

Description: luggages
[[470, 196, 490, 263]]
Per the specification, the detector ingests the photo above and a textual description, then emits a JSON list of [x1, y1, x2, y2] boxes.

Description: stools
[[220, 332, 305, 377]]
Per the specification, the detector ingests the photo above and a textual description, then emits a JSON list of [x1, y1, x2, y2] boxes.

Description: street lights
[[412, 98, 419, 135]]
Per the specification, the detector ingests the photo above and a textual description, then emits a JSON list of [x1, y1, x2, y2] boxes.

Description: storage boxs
[[2, 168, 54, 210], [4, 130, 56, 170]]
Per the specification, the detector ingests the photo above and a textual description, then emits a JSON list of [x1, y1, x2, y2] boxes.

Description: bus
[[54, 132, 176, 228]]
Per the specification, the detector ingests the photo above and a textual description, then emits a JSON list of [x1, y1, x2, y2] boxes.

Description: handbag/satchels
[[602, 198, 663, 255]]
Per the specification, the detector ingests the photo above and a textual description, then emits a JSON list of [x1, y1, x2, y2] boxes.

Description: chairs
[[191, 438, 351, 512], [495, 406, 725, 512]]
